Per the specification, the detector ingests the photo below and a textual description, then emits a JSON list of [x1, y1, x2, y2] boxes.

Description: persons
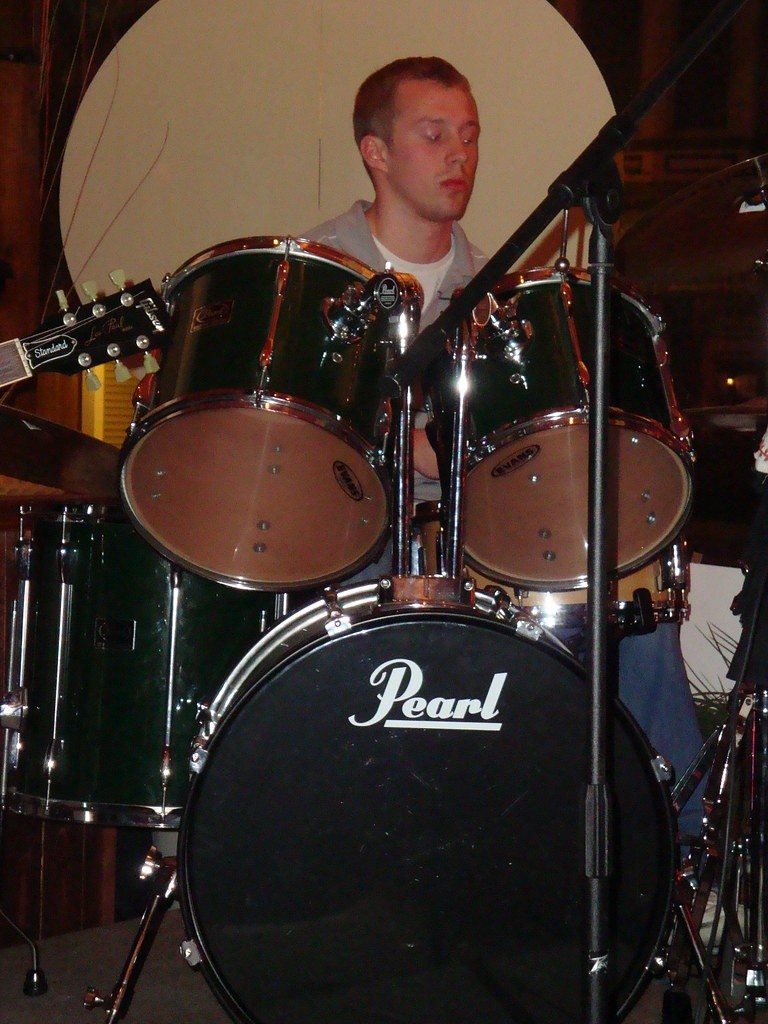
[[298, 56, 747, 955]]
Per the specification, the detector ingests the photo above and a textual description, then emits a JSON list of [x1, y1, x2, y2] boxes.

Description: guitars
[[0, 269, 173, 393]]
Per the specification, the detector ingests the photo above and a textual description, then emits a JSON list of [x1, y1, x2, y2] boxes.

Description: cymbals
[[0, 402, 126, 498], [612, 153, 767, 290], [681, 393, 768, 434]]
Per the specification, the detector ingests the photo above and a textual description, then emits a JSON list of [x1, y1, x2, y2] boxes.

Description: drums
[[173, 578, 680, 1022], [122, 232, 428, 594], [6, 500, 272, 827], [421, 263, 698, 592]]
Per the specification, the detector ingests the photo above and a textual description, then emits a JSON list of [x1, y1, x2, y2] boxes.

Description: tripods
[[670, 559, 768, 1024]]
